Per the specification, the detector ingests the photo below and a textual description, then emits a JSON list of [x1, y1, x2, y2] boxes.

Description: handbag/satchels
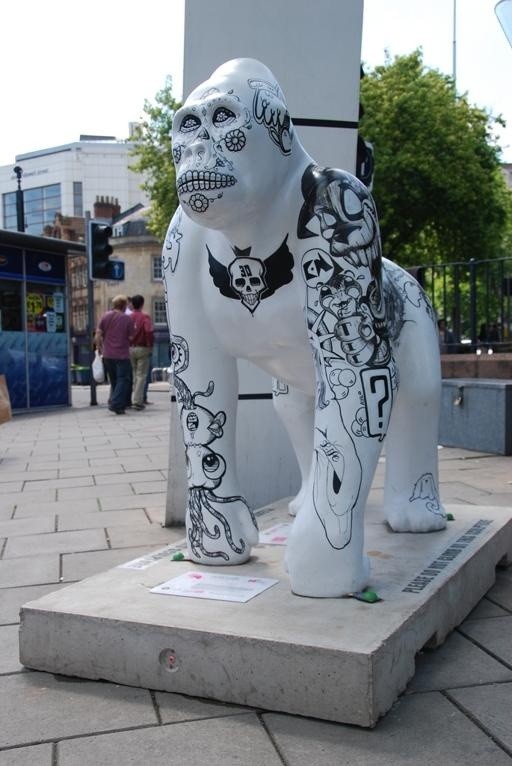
[[92, 348, 106, 384]]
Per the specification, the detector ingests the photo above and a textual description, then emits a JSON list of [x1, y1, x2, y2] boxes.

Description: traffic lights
[[89, 220, 114, 282]]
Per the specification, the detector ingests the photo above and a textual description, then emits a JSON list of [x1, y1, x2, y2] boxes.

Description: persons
[[436, 317, 511, 355], [108, 296, 136, 407], [128, 294, 155, 410], [95, 294, 136, 414], [142, 345, 155, 405]]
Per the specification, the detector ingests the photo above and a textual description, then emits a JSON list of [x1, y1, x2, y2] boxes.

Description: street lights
[[13, 166, 24, 232]]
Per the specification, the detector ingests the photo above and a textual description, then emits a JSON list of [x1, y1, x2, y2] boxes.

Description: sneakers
[[107, 398, 145, 414]]
